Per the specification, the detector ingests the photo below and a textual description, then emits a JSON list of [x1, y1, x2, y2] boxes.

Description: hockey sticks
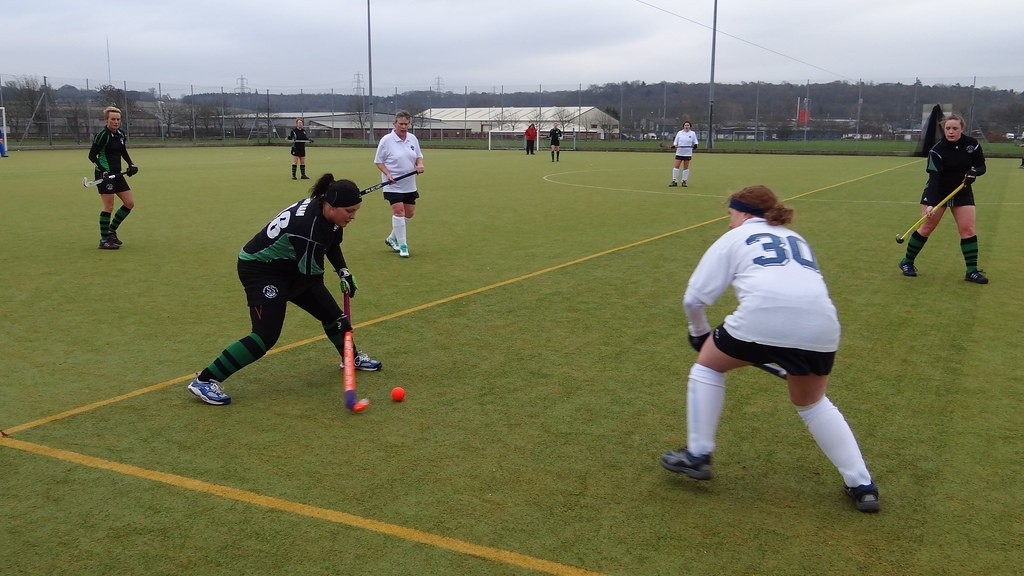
[[342, 288, 369, 412], [82, 170, 127, 188], [660, 143, 694, 148], [359, 171, 417, 197], [750, 362, 787, 379], [896, 183, 964, 244], [285, 137, 311, 142]]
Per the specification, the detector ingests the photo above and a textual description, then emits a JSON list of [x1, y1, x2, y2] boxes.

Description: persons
[[374, 111, 424, 258], [287, 119, 314, 180], [547, 123, 562, 162], [88, 106, 138, 250], [187, 174, 382, 406], [525, 124, 537, 155], [659, 185, 880, 512], [0, 130, 8, 157], [669, 121, 699, 187], [898, 114, 988, 284]]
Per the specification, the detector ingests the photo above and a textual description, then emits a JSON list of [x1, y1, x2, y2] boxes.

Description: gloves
[[103, 171, 112, 184], [128, 164, 138, 177], [962, 170, 976, 187], [686, 331, 712, 352], [288, 136, 291, 139], [339, 269, 357, 299], [692, 145, 697, 150], [309, 139, 313, 144], [337, 314, 353, 334], [671, 146, 675, 149]]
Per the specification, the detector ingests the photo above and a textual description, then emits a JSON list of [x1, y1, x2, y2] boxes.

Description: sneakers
[[846, 482, 880, 511], [385, 236, 400, 252], [669, 179, 677, 187], [98, 239, 119, 249], [899, 260, 917, 277], [399, 244, 409, 258], [339, 350, 382, 371], [187, 377, 231, 405], [681, 180, 687, 187], [964, 271, 989, 285], [659, 447, 713, 480], [110, 232, 122, 245]]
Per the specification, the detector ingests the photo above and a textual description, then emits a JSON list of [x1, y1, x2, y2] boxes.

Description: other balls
[[391, 387, 405, 401]]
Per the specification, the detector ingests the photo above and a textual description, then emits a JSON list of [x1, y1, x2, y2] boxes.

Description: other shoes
[[300, 175, 309, 179], [292, 176, 297, 180]]
